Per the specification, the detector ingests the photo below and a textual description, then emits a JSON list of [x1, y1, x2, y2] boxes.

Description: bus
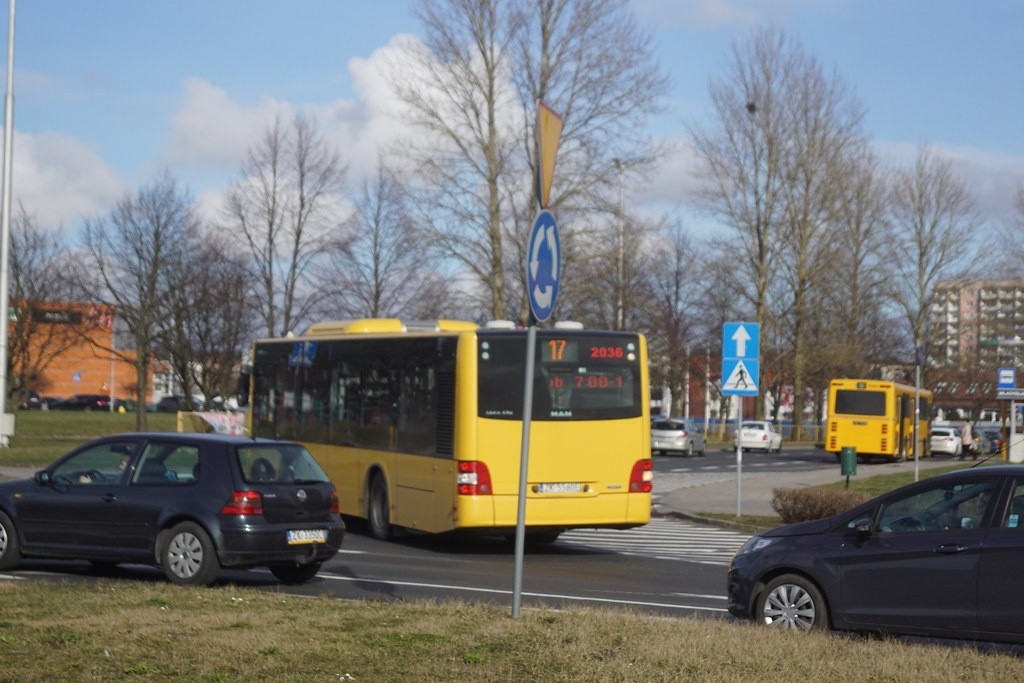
[[238, 316, 655, 554], [826, 378, 934, 462]]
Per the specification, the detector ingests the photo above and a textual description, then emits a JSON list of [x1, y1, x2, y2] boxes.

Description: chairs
[[138, 454, 282, 487], [1007, 494, 1024, 527]]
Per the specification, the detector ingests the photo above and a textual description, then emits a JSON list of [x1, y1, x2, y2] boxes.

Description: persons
[[955, 418, 976, 460]]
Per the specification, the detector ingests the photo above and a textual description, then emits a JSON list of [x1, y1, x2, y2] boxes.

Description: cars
[[725, 464, 1024, 656], [0, 432, 345, 586], [66, 394, 112, 412], [983, 430, 1005, 456], [734, 420, 783, 453], [39, 396, 64, 412], [651, 418, 708, 458], [966, 430, 991, 456], [17, 390, 49, 411], [158, 394, 207, 415], [930, 427, 966, 458]]
